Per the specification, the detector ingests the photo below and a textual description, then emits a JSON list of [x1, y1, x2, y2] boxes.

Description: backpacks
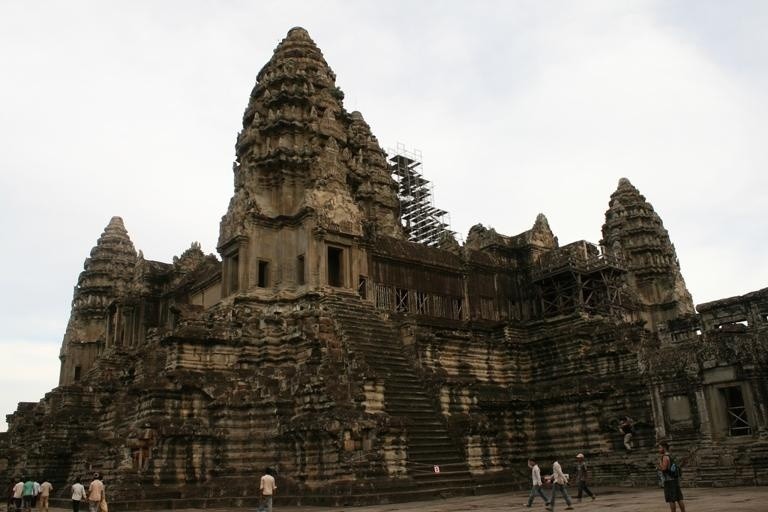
[[660, 454, 683, 477]]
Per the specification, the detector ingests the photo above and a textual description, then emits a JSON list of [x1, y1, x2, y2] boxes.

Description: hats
[[576, 452, 584, 457]]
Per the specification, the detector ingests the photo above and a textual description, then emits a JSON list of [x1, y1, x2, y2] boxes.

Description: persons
[[654, 456, 664, 489], [542, 457, 573, 512], [86, 472, 103, 512], [616, 417, 634, 455], [522, 457, 551, 510], [258, 466, 277, 512], [575, 452, 595, 504], [5, 477, 54, 512], [656, 441, 686, 512], [69, 476, 86, 512], [98, 475, 108, 512]]
